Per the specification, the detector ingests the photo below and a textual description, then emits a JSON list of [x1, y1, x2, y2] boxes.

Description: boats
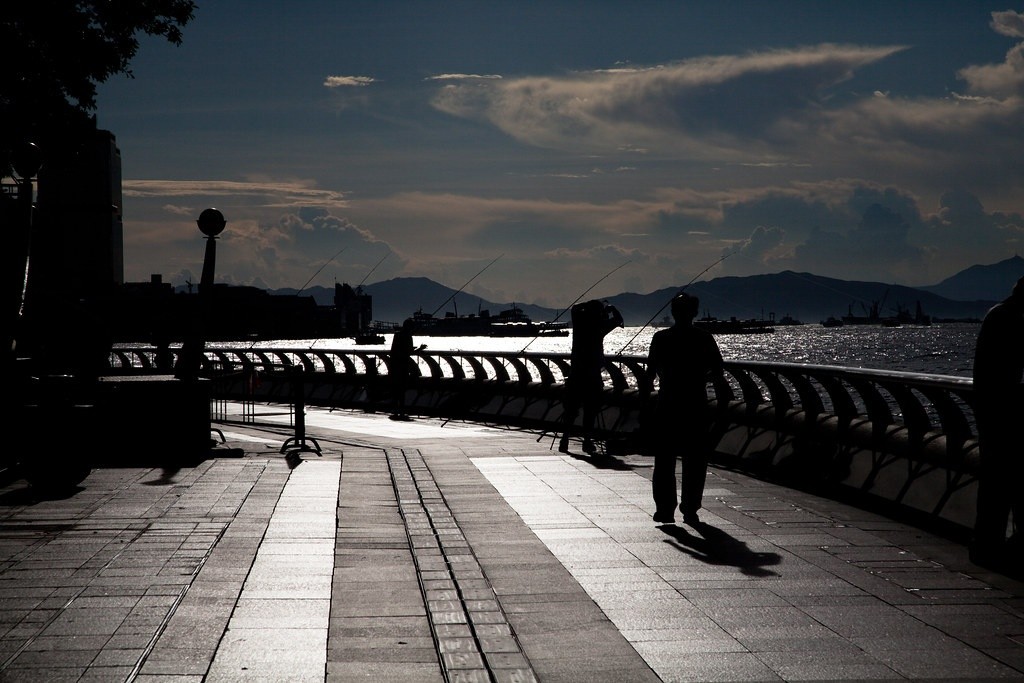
[[881, 319, 900, 327], [695, 312, 805, 334], [649, 316, 674, 326], [374, 300, 570, 337], [354, 323, 385, 345], [822, 316, 843, 329]]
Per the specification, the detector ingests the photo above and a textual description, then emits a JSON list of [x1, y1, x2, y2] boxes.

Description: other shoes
[[559, 440, 568, 452], [684, 514, 699, 526], [653, 510, 675, 523], [969, 546, 1024, 576], [582, 440, 597, 452]]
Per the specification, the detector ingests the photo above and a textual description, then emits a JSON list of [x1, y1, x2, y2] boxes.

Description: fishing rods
[[234, 255, 335, 365], [326, 251, 507, 416], [534, 245, 743, 444], [437, 255, 632, 429], [264, 247, 395, 407]]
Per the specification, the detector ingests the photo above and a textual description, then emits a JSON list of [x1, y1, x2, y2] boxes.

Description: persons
[[559, 300, 624, 453], [969, 276, 1024, 565], [389, 318, 427, 421], [639, 292, 724, 524]]
